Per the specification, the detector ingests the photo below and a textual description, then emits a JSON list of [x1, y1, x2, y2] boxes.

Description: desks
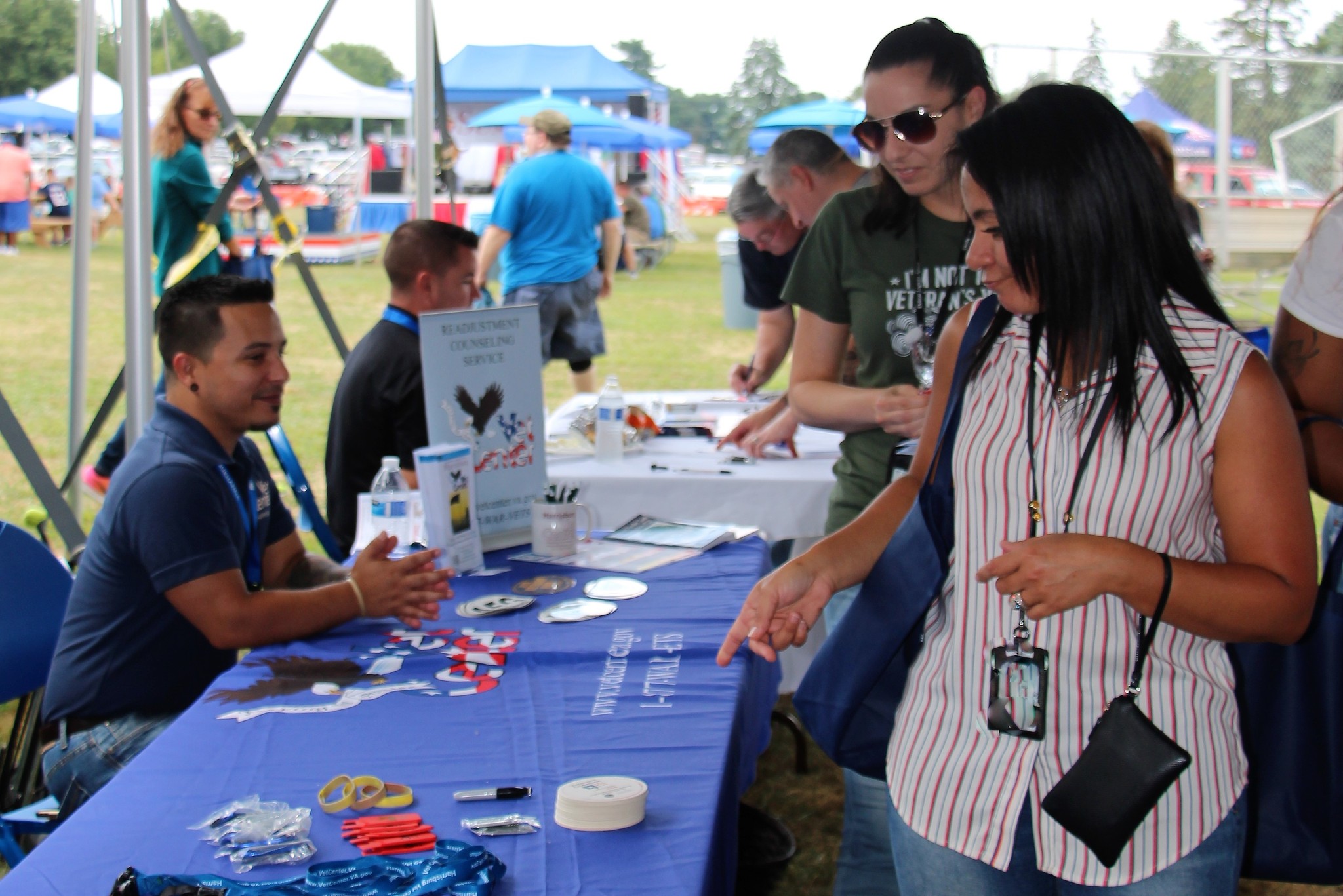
[[352, 194, 494, 235], [0, 529, 782, 896], [541, 388, 846, 772]]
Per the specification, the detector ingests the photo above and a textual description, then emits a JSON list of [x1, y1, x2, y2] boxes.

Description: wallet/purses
[[1038, 690, 1190, 871]]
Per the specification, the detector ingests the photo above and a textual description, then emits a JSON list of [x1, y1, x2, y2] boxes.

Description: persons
[[714, 80, 1319, 895], [34, 170, 126, 247], [321, 219, 480, 550], [469, 112, 624, 396], [1130, 118, 1216, 286], [715, 126, 885, 460], [785, 14, 1003, 894], [35, 275, 455, 808], [83, 76, 252, 504], [0, 134, 34, 257], [1271, 182, 1343, 503]]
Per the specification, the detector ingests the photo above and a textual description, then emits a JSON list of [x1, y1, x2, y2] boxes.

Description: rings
[[753, 438, 760, 444]]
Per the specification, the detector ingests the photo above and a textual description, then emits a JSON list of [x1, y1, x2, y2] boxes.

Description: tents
[[146, 18, 416, 192], [37, 70, 124, 116], [1109, 88, 1255, 159], [387, 43, 681, 235]]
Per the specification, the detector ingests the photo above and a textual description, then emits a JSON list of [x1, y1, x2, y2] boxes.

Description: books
[[609, 513, 763, 552]]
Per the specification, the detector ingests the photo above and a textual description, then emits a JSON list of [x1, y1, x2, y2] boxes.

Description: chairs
[[0, 522, 75, 871]]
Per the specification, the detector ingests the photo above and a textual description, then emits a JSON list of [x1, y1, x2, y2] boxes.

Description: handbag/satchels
[[1225, 528, 1342, 886], [789, 294, 1013, 781]]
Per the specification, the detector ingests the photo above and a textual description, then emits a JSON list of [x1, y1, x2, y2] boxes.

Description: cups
[[531, 499, 592, 558]]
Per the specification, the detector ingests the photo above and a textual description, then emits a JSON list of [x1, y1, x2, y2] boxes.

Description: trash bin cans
[[305, 205, 336, 231], [716, 230, 758, 329]]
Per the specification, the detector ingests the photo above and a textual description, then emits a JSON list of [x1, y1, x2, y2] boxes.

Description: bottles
[[370, 456, 413, 563], [595, 376, 624, 467]]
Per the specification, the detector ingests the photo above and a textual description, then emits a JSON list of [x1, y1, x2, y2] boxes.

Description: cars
[[1177, 166, 1297, 197]]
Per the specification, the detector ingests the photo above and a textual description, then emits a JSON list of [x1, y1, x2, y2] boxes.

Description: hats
[[519, 110, 572, 138]]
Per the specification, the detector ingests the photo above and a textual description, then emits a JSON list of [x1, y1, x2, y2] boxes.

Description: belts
[[39, 704, 136, 745]]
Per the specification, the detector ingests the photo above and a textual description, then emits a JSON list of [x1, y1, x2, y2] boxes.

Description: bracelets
[[345, 578, 368, 618]]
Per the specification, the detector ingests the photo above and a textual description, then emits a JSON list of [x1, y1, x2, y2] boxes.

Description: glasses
[[739, 210, 784, 243], [851, 90, 967, 155], [181, 105, 221, 121]]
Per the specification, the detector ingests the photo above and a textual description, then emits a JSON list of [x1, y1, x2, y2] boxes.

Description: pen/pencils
[[454, 787, 533, 800], [744, 355, 756, 397], [543, 485, 579, 503], [715, 457, 749, 462], [652, 465, 730, 473]]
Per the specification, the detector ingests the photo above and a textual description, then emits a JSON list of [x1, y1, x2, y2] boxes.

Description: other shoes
[[0, 244, 19, 255], [82, 468, 112, 504]]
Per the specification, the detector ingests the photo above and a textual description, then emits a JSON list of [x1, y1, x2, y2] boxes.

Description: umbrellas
[[0, 96, 123, 140], [469, 95, 622, 128], [747, 97, 871, 156], [616, 114, 694, 150], [503, 117, 647, 150]]
